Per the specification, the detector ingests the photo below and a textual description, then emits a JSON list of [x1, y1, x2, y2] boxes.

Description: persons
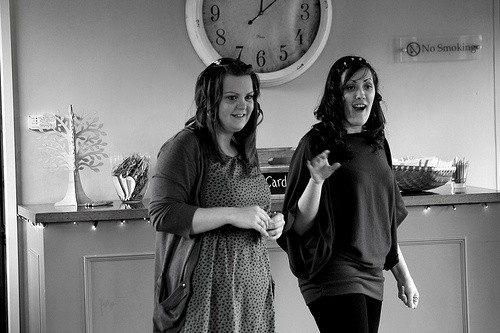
[[276, 55, 420, 333], [149, 57, 285, 333]]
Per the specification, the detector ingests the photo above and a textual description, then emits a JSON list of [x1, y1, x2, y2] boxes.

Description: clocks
[[185, 0, 333, 87]]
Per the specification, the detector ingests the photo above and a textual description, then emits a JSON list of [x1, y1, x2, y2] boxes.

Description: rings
[[260, 221, 265, 227]]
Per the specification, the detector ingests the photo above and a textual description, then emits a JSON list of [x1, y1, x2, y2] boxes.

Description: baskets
[[392, 156, 456, 190]]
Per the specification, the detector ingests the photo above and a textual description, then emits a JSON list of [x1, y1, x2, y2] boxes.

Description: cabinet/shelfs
[[17, 184, 500, 333]]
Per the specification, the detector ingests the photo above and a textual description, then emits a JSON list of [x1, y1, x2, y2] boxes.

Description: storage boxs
[[259, 166, 290, 200]]
